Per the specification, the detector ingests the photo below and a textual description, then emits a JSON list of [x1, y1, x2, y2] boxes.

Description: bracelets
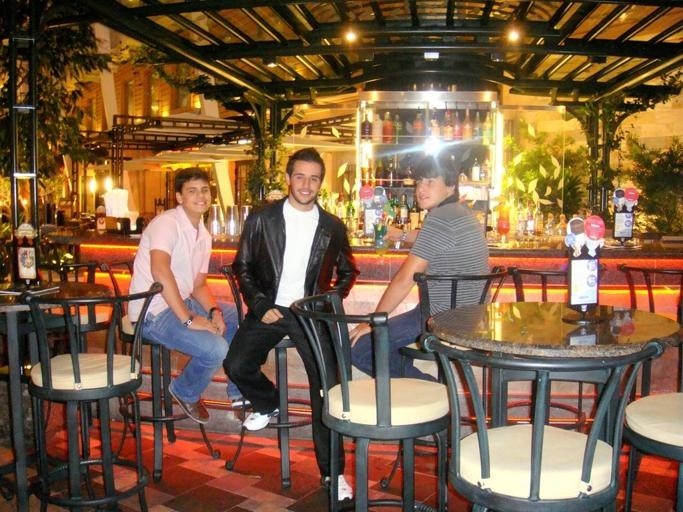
[[178, 313, 193, 328], [207, 306, 221, 319]]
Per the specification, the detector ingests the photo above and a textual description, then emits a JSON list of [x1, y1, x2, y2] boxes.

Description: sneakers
[[322, 475, 353, 503], [168, 380, 210, 425], [231, 397, 251, 407], [242, 407, 279, 432]]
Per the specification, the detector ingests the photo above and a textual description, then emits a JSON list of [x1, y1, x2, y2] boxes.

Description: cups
[[370, 222, 389, 251], [115, 217, 131, 235]]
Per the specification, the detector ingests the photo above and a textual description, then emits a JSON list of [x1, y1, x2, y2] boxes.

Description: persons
[[125, 165, 251, 423], [223, 148, 360, 504], [348, 155, 490, 382]]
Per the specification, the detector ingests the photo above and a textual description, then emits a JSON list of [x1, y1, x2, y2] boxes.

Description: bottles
[[361, 107, 493, 232], [317, 192, 360, 237], [95, 195, 107, 231], [135, 210, 146, 233], [495, 197, 579, 244], [43, 192, 79, 229], [207, 203, 252, 244]]
